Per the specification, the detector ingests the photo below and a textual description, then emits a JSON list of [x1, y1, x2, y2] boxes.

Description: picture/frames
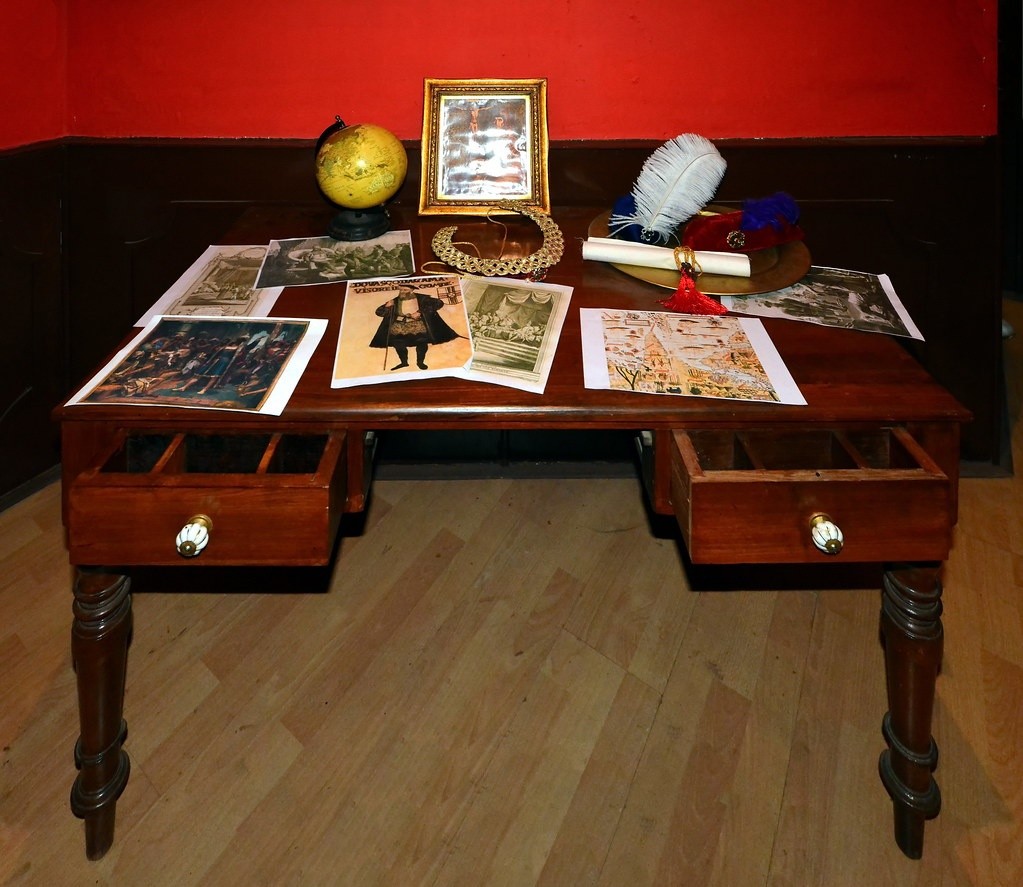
[[417, 77, 553, 220]]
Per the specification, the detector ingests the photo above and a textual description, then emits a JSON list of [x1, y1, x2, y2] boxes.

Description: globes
[[314, 114, 408, 242]]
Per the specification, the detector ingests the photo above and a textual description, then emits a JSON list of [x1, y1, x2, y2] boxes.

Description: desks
[[48, 200, 976, 866]]
